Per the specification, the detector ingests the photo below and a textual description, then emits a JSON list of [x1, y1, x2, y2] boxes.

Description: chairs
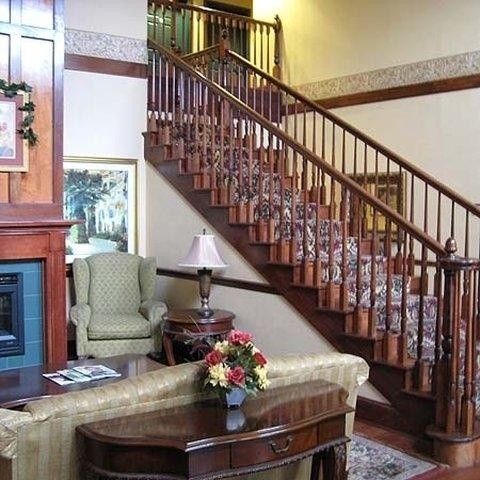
[[68, 252, 168, 366]]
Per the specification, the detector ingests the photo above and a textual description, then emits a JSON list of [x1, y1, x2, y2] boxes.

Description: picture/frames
[[62, 155, 141, 268], [340, 171, 409, 244], [1, 89, 31, 174]]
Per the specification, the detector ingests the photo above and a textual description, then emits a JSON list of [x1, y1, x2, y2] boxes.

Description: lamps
[[177, 226, 229, 317]]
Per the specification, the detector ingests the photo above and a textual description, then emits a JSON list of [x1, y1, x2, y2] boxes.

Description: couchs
[[1, 351, 370, 479]]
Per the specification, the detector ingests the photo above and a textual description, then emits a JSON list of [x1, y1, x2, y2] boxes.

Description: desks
[[1, 352, 171, 413], [162, 307, 236, 366], [73, 377, 360, 480]]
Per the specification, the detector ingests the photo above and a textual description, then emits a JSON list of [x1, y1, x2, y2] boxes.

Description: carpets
[[346, 428, 452, 480]]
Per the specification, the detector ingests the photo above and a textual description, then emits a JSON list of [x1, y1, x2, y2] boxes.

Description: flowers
[[178, 316, 272, 395]]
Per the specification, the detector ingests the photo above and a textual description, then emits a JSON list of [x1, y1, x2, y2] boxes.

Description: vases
[[215, 386, 250, 410], [225, 405, 247, 432]]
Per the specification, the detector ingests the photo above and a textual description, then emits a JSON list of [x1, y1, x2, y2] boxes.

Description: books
[[42, 364, 121, 386]]
